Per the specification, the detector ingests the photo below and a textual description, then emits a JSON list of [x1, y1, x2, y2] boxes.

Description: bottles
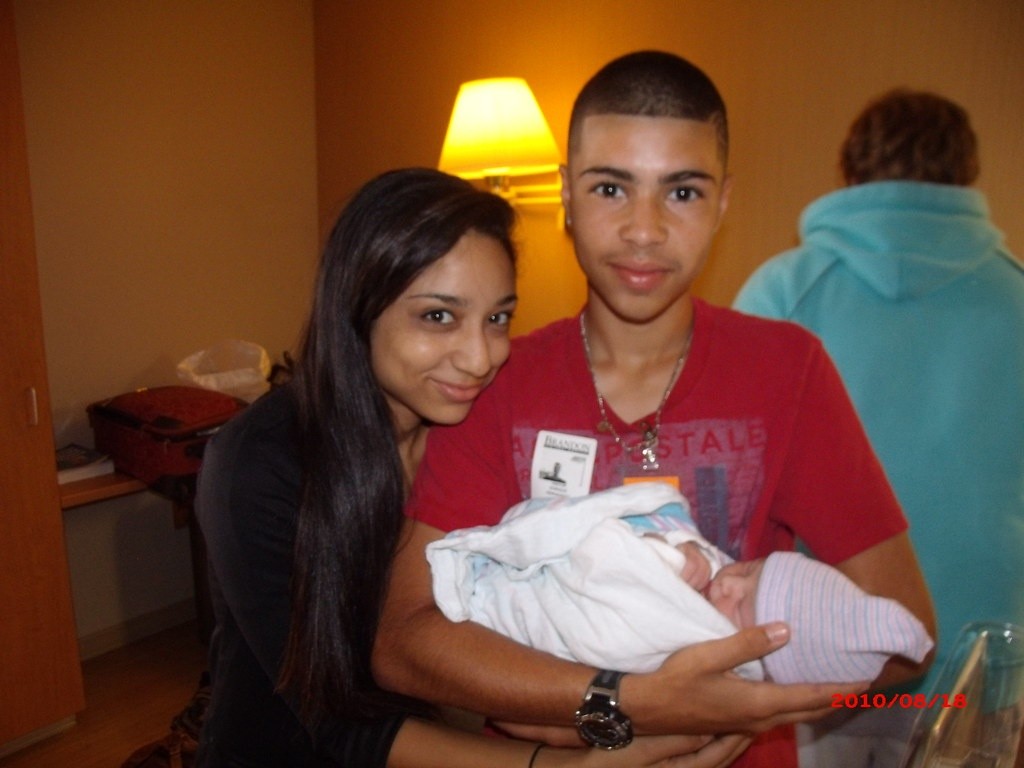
[[899, 622, 1024, 768]]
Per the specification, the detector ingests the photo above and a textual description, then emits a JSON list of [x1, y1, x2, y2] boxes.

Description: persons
[[543, 463, 566, 483], [194, 166, 753, 768], [370, 48, 938, 768], [732, 88, 1023, 768], [426, 480, 934, 684]]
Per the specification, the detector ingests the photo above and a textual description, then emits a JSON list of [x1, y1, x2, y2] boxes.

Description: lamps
[[437, 76, 566, 228]]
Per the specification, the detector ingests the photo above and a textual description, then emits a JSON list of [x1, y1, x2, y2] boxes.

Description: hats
[[754, 549, 934, 686]]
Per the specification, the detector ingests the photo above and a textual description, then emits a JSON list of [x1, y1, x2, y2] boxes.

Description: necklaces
[[581, 308, 695, 469]]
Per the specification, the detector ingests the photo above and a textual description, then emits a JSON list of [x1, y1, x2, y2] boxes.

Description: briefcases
[[84, 381, 252, 492]]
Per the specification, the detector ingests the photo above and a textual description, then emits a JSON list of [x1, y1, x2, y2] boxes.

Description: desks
[[59, 458, 216, 649]]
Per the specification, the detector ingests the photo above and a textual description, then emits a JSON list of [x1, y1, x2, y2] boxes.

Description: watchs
[[575, 670, 634, 749]]
